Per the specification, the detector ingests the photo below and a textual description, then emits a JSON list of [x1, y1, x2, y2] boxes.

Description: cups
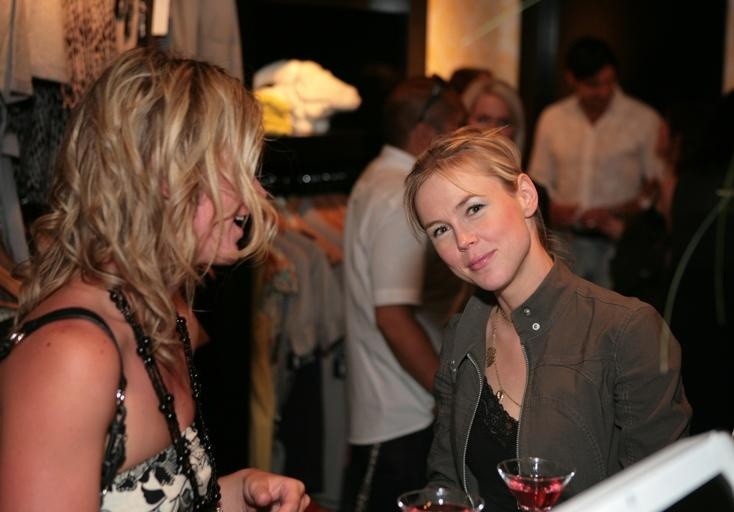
[[497, 456, 576, 512], [397, 489, 485, 512]]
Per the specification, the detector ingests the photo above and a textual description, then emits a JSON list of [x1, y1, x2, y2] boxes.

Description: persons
[[338, 70, 470, 511], [446, 66, 524, 161], [514, 34, 674, 287], [401, 123, 695, 511], [603, 93, 734, 438], [1, 48, 315, 512]]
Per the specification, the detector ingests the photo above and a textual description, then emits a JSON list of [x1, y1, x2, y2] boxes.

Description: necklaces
[[487, 303, 525, 411]]
